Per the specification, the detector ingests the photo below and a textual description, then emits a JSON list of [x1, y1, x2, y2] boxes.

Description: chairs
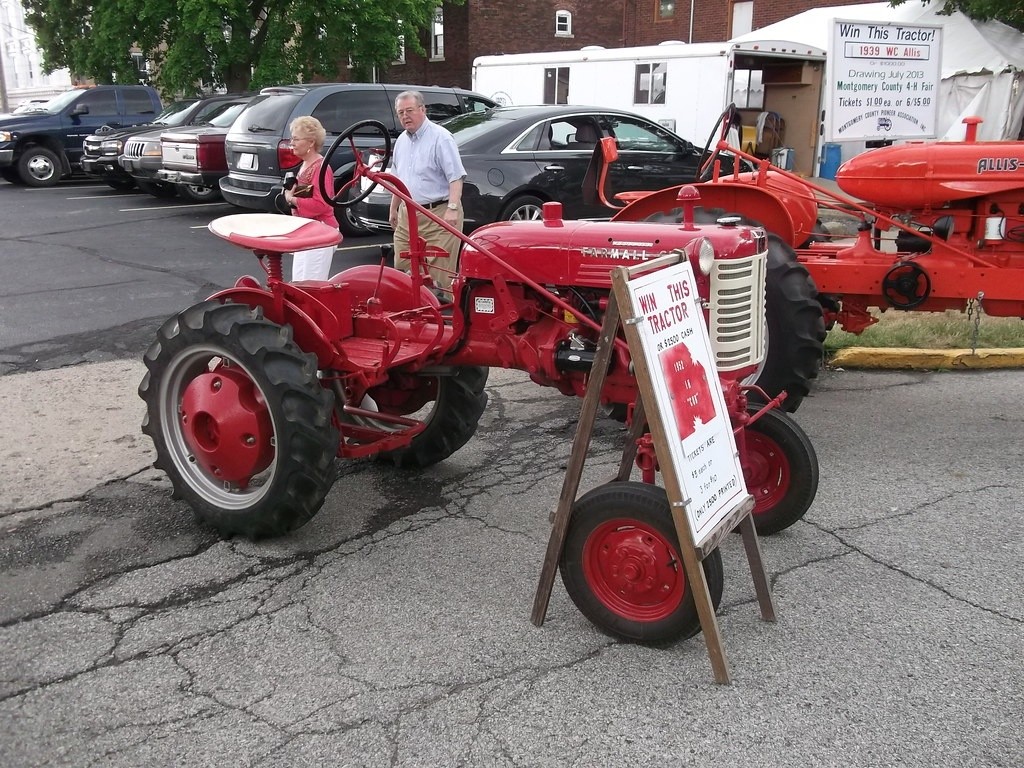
[[594, 137, 659, 211], [531, 124, 556, 149], [568, 127, 597, 149]]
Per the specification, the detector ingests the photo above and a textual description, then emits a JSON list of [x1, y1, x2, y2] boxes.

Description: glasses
[[289, 137, 309, 142], [397, 106, 420, 117]]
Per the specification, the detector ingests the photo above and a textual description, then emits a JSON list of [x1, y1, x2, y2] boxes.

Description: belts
[[421, 200, 448, 210]]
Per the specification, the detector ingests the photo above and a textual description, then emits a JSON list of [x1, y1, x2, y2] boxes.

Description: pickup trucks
[[0, 86, 161, 189]]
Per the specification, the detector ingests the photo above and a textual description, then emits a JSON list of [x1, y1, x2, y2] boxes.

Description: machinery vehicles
[[580, 99, 1020, 344], [136, 116, 821, 652]]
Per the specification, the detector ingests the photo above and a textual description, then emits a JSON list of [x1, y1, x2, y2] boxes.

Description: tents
[[730, 0, 1024, 147]]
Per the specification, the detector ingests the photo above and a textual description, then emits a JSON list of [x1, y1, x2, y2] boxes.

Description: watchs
[[448, 203, 458, 209]]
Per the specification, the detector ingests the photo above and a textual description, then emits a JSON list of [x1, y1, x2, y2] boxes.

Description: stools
[[207, 212, 342, 289]]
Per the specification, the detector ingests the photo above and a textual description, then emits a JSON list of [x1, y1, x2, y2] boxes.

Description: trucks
[[464, 38, 771, 156]]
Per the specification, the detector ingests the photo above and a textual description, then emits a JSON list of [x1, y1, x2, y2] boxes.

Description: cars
[[116, 94, 260, 201], [79, 94, 250, 196], [216, 82, 505, 239], [156, 91, 257, 189], [351, 105, 753, 234]]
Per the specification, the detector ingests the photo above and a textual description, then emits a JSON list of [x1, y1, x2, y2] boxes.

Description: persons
[[284, 116, 340, 281], [388, 90, 468, 300]]
